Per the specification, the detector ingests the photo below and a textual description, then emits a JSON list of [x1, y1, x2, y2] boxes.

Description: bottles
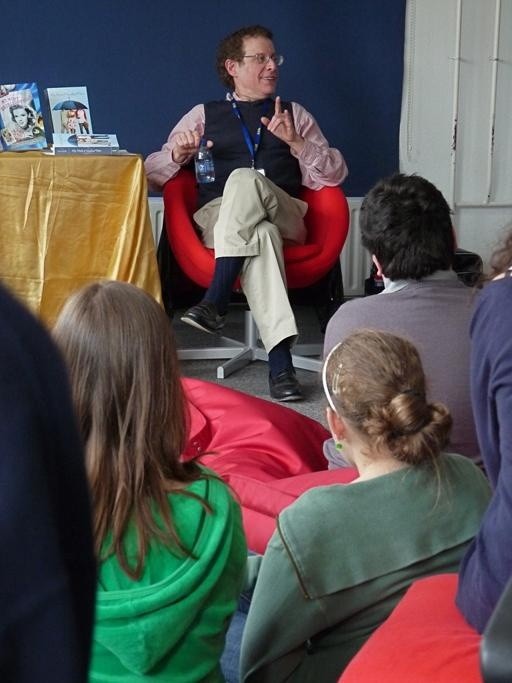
[[195, 136, 216, 184]]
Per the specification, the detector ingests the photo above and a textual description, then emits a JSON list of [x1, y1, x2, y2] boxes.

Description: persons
[[143, 24, 350, 402], [321, 173, 484, 471], [1, 282, 99, 682], [50, 279, 248, 683], [218, 328, 494, 682], [454, 224, 512, 634]]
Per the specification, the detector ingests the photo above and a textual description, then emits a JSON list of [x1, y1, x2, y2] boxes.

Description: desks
[[1, 149, 165, 358]]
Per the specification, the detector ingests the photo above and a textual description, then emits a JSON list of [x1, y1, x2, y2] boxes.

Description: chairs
[[339, 570, 483, 683], [162, 167, 349, 381]]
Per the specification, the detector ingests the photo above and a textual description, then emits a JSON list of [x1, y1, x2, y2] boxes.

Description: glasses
[[232, 51, 284, 67]]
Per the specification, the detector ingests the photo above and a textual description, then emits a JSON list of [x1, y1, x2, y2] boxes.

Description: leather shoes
[[180, 301, 228, 336], [268, 363, 304, 401]]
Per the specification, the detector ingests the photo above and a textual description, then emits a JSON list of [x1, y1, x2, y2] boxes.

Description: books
[[0, 82, 120, 156]]
